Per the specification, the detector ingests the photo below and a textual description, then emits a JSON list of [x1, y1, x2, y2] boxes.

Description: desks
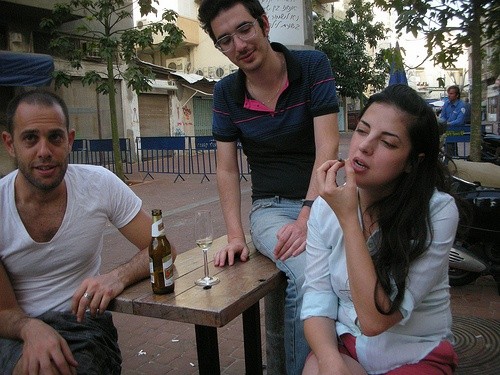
[[88, 234, 286, 375]]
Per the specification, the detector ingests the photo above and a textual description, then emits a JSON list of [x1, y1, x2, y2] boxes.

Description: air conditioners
[[212, 63, 233, 79], [136, 19, 148, 31], [166, 57, 187, 73], [194, 66, 212, 78]]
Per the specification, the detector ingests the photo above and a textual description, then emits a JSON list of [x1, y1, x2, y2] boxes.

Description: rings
[[83, 293, 92, 301]]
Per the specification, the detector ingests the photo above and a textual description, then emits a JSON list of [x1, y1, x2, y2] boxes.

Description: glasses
[[214, 18, 258, 53]]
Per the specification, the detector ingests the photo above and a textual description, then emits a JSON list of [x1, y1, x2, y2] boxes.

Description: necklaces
[[242, 52, 288, 104]]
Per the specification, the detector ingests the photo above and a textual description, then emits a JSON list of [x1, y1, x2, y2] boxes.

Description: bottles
[[148, 209, 174, 294]]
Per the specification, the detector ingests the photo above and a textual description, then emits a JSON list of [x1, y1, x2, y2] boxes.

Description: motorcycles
[[424, 151, 500, 288]]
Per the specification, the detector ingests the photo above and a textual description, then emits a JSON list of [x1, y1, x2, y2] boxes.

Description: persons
[[301, 84, 459, 375], [462, 96, 471, 125], [0, 87, 178, 375], [195, 0, 349, 374], [438, 85, 467, 158]]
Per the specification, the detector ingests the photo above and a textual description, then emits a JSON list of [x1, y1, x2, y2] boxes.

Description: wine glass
[[195, 210, 220, 285]]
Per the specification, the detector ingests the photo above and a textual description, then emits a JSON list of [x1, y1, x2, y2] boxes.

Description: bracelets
[[302, 200, 315, 208]]
